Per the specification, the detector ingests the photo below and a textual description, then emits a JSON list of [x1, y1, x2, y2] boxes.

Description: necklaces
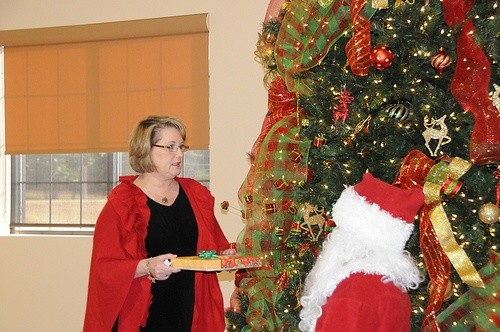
[[136, 179, 178, 204]]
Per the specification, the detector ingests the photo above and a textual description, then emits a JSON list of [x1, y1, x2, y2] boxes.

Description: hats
[[329, 174, 425, 252]]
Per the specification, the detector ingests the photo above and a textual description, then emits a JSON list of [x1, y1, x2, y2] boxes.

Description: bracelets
[[145, 258, 157, 283]]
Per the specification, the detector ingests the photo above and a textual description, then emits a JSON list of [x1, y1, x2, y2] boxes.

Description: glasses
[[152, 143, 190, 153]]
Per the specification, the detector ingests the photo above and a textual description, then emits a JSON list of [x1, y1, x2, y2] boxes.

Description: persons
[[83, 114, 236, 332], [301, 172, 424, 332]]
